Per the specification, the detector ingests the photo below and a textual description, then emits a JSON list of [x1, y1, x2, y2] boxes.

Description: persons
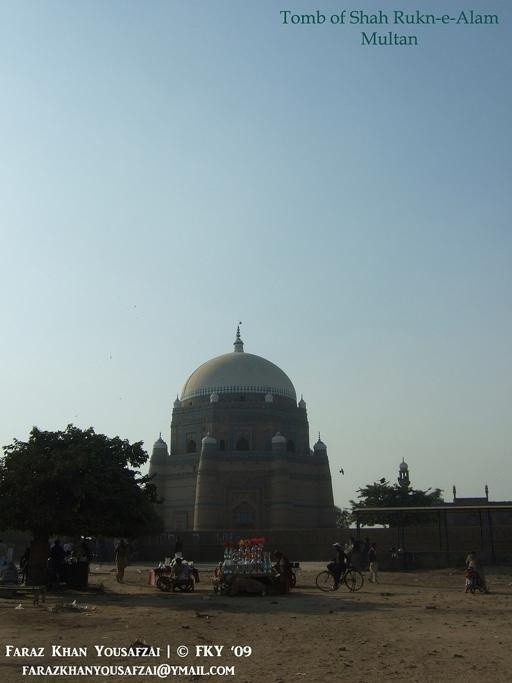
[[367, 541, 379, 585], [173, 535, 184, 555], [270, 550, 294, 597], [169, 558, 196, 592], [329, 541, 348, 589], [463, 550, 486, 594], [114, 538, 130, 583], [20, 537, 95, 587]]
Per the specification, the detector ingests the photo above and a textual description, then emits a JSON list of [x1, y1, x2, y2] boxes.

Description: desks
[[0, 585, 46, 605]]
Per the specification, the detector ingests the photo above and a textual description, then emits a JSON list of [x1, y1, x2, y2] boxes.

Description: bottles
[[224, 544, 272, 574]]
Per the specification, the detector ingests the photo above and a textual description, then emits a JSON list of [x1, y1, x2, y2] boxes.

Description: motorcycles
[[463, 566, 480, 594]]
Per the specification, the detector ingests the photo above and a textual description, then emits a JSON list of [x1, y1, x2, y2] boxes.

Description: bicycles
[[315, 565, 365, 592]]
